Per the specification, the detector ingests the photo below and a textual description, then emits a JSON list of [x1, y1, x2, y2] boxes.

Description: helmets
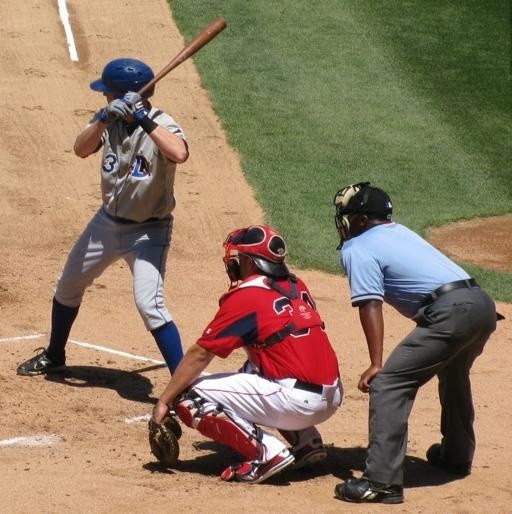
[[220, 225, 294, 278], [87, 57, 158, 99], [335, 181, 393, 222]]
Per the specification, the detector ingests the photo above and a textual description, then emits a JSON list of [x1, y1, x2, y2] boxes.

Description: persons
[[15, 57, 191, 379], [331, 181, 498, 505], [147, 222, 346, 484]]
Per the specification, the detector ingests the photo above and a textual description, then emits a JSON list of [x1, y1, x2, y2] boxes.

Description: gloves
[[97, 98, 135, 124], [121, 90, 148, 122]]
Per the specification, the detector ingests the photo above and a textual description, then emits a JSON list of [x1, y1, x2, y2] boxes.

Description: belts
[[416, 277, 479, 311], [293, 380, 323, 396]]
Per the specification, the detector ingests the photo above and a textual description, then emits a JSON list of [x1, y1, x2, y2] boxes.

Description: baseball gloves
[[149, 407, 182, 466]]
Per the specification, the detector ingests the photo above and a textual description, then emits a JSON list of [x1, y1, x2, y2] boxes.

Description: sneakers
[[333, 475, 405, 505], [234, 447, 297, 485], [287, 438, 328, 474], [16, 345, 68, 377], [425, 442, 474, 477]]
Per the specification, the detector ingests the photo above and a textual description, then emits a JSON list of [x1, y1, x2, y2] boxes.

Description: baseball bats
[[109, 16, 227, 120]]
